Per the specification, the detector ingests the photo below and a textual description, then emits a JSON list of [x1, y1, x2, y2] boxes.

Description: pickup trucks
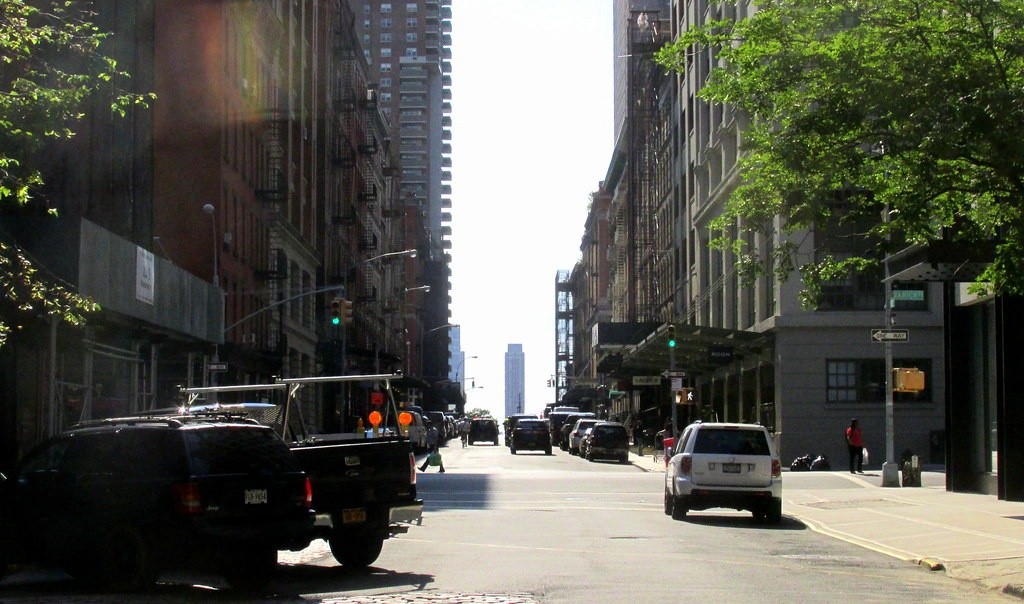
[[165, 400, 424, 578]]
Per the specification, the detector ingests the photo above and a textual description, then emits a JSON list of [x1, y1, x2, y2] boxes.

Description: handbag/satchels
[[427, 450, 441, 466]]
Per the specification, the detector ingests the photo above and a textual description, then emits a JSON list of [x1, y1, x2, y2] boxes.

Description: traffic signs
[[871, 329, 908, 343]]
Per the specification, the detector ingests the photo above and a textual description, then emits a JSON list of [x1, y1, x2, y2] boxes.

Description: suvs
[[568, 418, 605, 455], [5, 409, 314, 589], [585, 421, 629, 463], [559, 412, 597, 451], [662, 418, 783, 525]]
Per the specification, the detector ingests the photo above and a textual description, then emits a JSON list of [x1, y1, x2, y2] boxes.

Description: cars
[[360, 405, 580, 455], [579, 426, 594, 457]]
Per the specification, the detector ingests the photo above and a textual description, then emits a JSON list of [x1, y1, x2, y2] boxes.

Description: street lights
[[202, 202, 225, 391], [338, 246, 480, 411]]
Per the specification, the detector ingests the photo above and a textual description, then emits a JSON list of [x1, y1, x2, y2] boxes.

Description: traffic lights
[[344, 299, 353, 326], [331, 299, 341, 326]]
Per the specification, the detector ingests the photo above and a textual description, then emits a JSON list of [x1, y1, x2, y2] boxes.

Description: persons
[[843, 418, 863, 475], [457, 417, 470, 447], [417, 420, 445, 474], [633, 421, 647, 458]]
